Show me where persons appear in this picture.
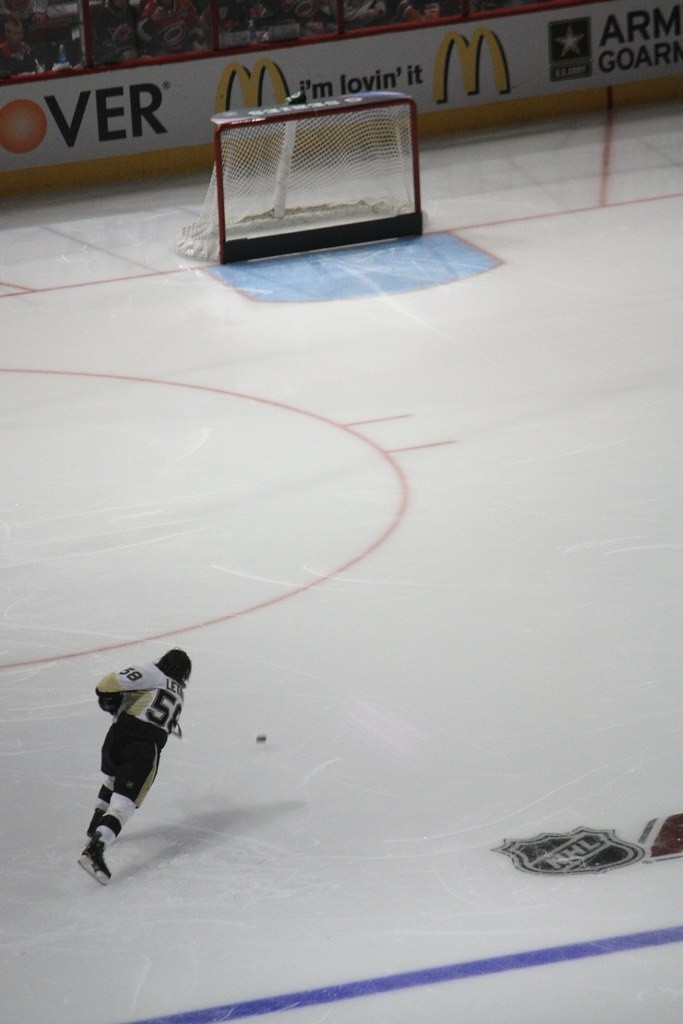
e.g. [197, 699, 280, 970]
[0, 0, 438, 76]
[78, 649, 192, 886]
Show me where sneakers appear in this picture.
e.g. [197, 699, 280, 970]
[77, 831, 112, 886]
[87, 808, 106, 838]
